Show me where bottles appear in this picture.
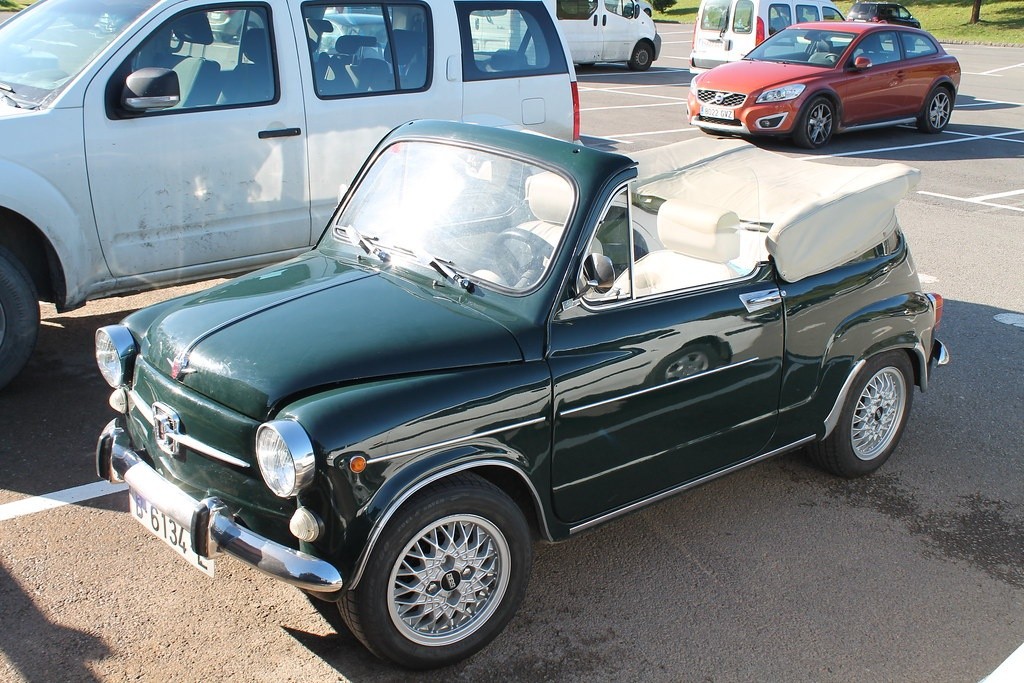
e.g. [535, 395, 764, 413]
[704, 15, 709, 27]
[738, 19, 742, 26]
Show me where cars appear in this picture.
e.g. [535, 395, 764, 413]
[307, 5, 392, 62]
[686, 21, 962, 150]
[205, 8, 263, 44]
[94, 119, 949, 671]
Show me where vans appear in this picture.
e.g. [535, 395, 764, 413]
[690, 0, 854, 74]
[469, 0, 662, 72]
[0, 1, 579, 398]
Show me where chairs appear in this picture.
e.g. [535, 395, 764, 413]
[154, 13, 222, 108]
[807, 40, 837, 65]
[606, 195, 742, 299]
[223, 26, 277, 103]
[854, 33, 884, 65]
[500, 172, 603, 281]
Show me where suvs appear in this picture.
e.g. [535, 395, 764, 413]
[846, 1, 922, 29]
